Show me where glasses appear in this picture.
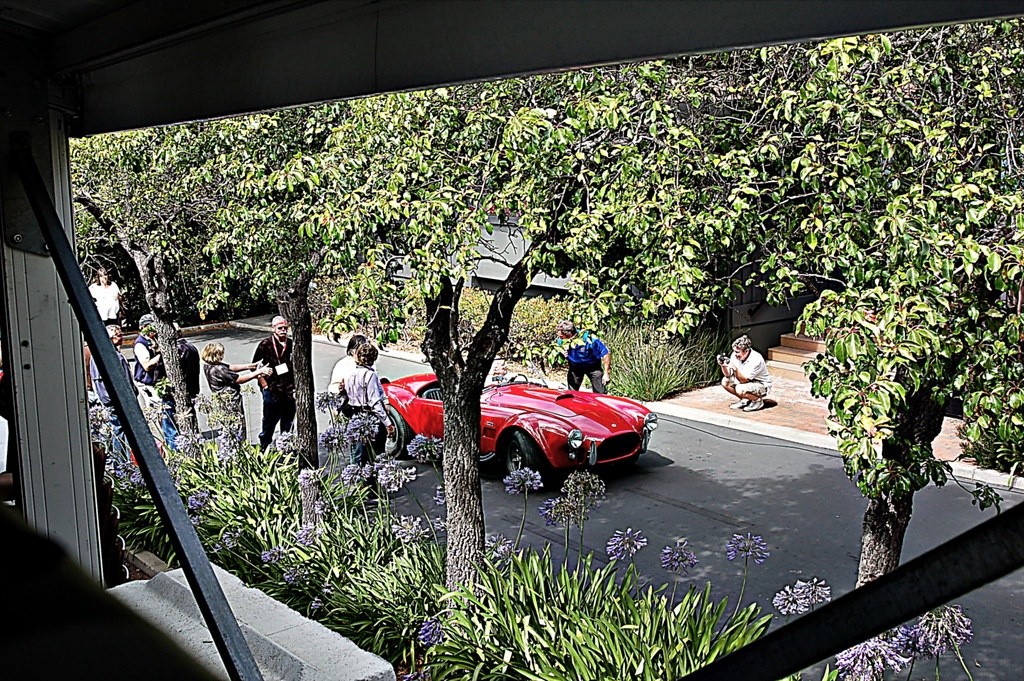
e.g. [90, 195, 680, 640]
[277, 326, 288, 329]
[556, 333, 564, 338]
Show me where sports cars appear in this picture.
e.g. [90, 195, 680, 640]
[379, 371, 658, 490]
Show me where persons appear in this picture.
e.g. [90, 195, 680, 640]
[557, 321, 610, 395]
[327, 334, 392, 414]
[88, 325, 139, 482]
[154, 323, 200, 451]
[250, 317, 297, 451]
[717, 336, 772, 412]
[87, 267, 123, 329]
[201, 342, 273, 441]
[341, 343, 397, 505]
[133, 313, 164, 445]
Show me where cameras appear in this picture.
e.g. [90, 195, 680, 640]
[718, 353, 730, 365]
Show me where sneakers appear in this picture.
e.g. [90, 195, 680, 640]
[729, 399, 750, 409]
[743, 398, 764, 412]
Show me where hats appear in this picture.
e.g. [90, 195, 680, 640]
[106, 325, 125, 339]
[139, 314, 155, 325]
[557, 322, 576, 332]
[272, 316, 288, 328]
[733, 335, 751, 350]
